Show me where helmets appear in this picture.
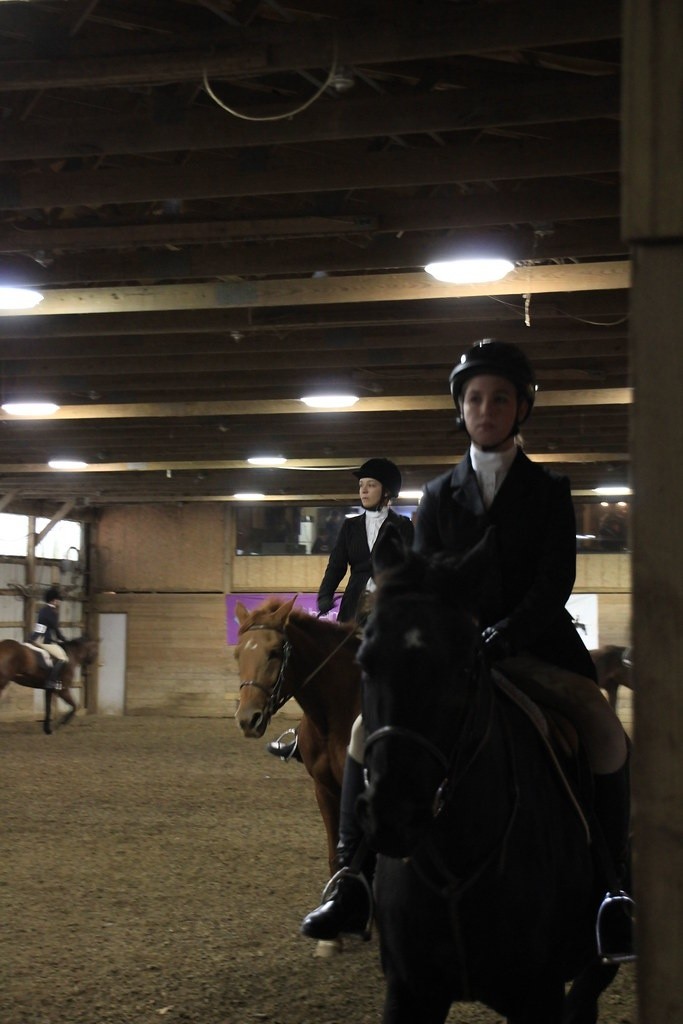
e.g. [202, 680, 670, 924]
[450, 339, 537, 421]
[46, 588, 62, 602]
[353, 458, 401, 497]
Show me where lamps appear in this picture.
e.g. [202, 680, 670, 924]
[1, 399, 61, 416]
[422, 257, 518, 285]
[0, 286, 44, 310]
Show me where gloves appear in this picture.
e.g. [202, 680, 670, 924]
[481, 626, 512, 658]
[319, 595, 333, 614]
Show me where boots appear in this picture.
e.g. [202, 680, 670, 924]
[590, 749, 637, 950]
[299, 747, 377, 940]
[268, 738, 303, 764]
[47, 659, 67, 690]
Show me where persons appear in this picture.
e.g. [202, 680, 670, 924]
[32, 587, 74, 691]
[269, 460, 415, 765]
[302, 336, 637, 959]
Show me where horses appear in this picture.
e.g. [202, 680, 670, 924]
[355, 519, 634, 1024]
[0, 635, 102, 735]
[233, 593, 366, 958]
